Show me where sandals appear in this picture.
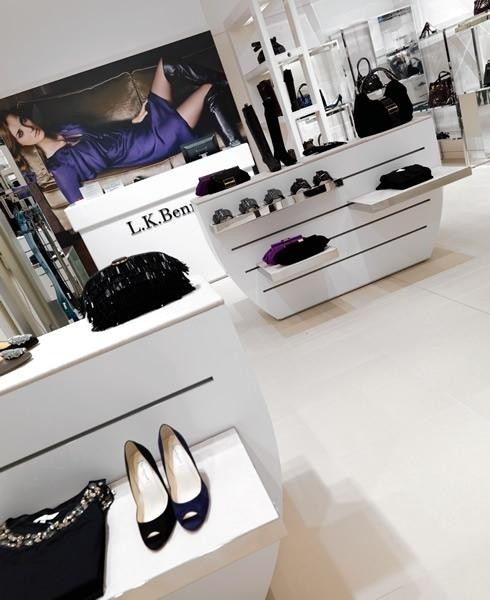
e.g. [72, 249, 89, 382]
[123, 439, 175, 550]
[157, 424, 208, 531]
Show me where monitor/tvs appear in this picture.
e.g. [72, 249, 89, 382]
[179, 132, 221, 163]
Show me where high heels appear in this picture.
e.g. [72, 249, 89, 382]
[419, 22, 437, 39]
[325, 94, 343, 111]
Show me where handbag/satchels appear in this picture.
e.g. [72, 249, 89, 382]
[80, 252, 196, 332]
[376, 164, 433, 190]
[474, 0, 489, 15]
[429, 77, 459, 108]
[263, 235, 330, 266]
[296, 89, 327, 118]
[354, 79, 413, 138]
[195, 166, 250, 197]
[258, 41, 285, 64]
[0, 479, 105, 598]
[484, 63, 490, 86]
[303, 142, 344, 156]
[287, 139, 315, 162]
[408, 60, 423, 77]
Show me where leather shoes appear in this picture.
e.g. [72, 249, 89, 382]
[0, 333, 39, 377]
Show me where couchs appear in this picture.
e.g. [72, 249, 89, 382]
[16, 47, 247, 235]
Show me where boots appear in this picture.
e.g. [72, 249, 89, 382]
[241, 105, 297, 172]
[203, 84, 244, 147]
[161, 52, 231, 97]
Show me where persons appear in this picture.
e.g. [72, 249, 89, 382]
[1, 53, 240, 206]
